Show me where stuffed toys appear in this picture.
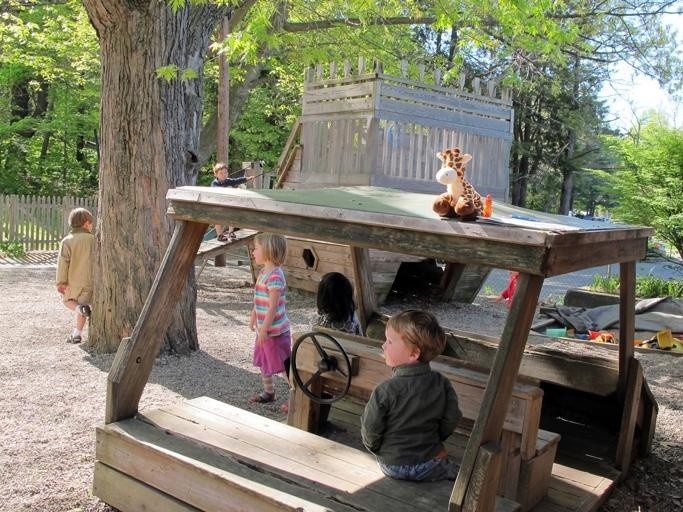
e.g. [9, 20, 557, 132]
[435, 147, 493, 218]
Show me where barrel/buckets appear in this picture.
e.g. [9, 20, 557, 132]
[545, 325, 568, 338]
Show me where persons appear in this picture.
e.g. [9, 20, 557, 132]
[360, 310, 463, 483]
[250, 232, 294, 412]
[210, 162, 254, 240]
[56, 207, 95, 343]
[283, 272, 364, 393]
[495, 267, 520, 311]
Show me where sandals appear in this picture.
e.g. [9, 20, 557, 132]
[229, 232, 236, 239]
[67, 334, 82, 344]
[252, 389, 276, 403]
[217, 234, 228, 241]
[79, 304, 90, 317]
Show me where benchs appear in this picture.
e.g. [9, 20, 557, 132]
[95, 394, 523, 511]
[286, 324, 560, 511]
